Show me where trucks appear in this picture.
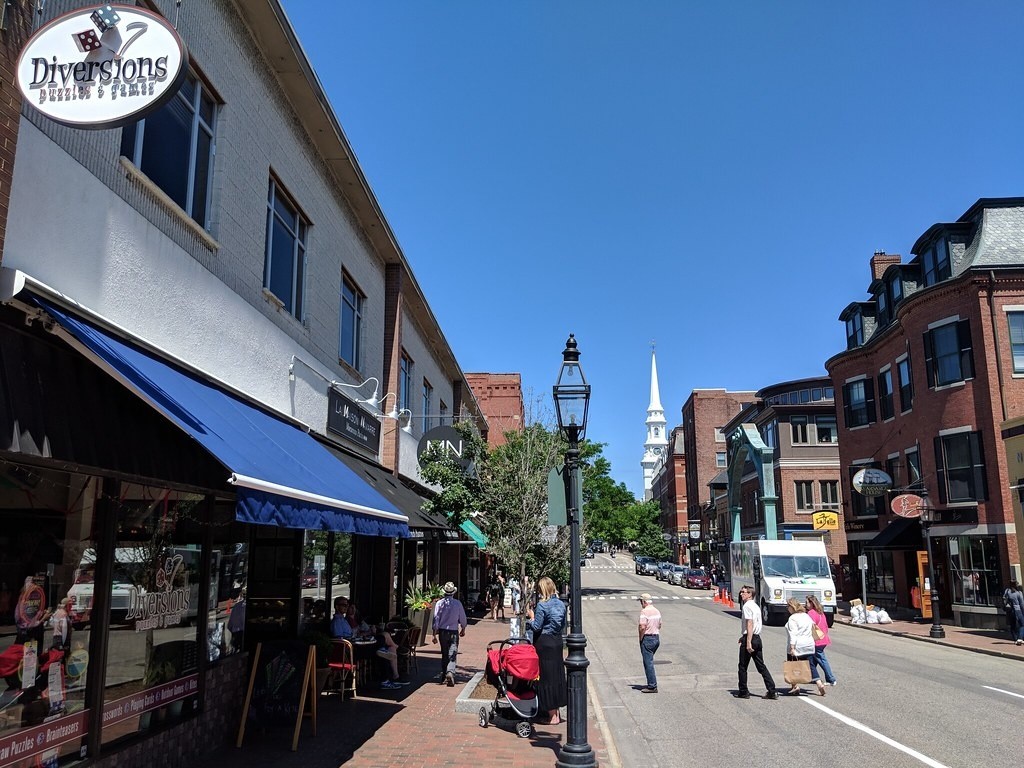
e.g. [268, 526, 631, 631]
[730, 539, 837, 628]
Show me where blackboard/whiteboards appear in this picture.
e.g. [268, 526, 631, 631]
[242, 642, 317, 717]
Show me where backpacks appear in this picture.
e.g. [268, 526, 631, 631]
[1003, 588, 1013, 612]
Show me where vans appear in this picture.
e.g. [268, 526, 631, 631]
[65, 546, 245, 632]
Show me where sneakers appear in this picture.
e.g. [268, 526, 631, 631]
[396, 647, 410, 655]
[392, 677, 410, 685]
[376, 648, 393, 660]
[381, 680, 402, 689]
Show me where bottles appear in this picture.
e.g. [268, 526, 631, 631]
[371, 625, 377, 634]
[351, 630, 355, 639]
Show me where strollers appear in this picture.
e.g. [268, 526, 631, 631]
[479, 637, 541, 738]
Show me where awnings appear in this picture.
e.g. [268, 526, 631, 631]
[317, 440, 460, 540]
[31, 293, 411, 539]
[446, 511, 490, 548]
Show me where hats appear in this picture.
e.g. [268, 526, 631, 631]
[637, 593, 652, 601]
[60, 597, 71, 608]
[442, 582, 457, 594]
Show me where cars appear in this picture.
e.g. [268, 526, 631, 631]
[681, 569, 712, 590]
[580, 538, 604, 567]
[667, 564, 689, 585]
[633, 552, 677, 581]
[302, 561, 345, 588]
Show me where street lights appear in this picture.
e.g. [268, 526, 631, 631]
[551, 332, 592, 768]
[917, 484, 945, 638]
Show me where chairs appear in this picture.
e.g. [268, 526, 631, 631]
[324, 637, 356, 702]
[385, 620, 421, 674]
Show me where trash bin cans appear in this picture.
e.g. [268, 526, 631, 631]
[718, 582, 730, 598]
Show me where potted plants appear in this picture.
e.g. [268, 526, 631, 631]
[402, 577, 432, 646]
[422, 582, 446, 634]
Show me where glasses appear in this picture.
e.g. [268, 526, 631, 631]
[337, 604, 349, 607]
[739, 590, 748, 593]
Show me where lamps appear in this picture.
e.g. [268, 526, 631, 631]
[373, 408, 413, 436]
[330, 376, 379, 408]
[355, 392, 398, 420]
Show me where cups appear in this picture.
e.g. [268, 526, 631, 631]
[379, 623, 385, 632]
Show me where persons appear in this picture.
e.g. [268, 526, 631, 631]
[509, 574, 521, 615]
[432, 582, 467, 687]
[732, 586, 779, 700]
[345, 600, 410, 685]
[228, 586, 247, 652]
[232, 576, 243, 602]
[805, 595, 836, 686]
[331, 596, 402, 688]
[784, 597, 826, 696]
[711, 564, 718, 584]
[1002, 578, 1024, 646]
[525, 577, 568, 725]
[700, 564, 704, 570]
[636, 593, 662, 693]
[53, 598, 74, 654]
[15, 575, 52, 656]
[301, 597, 325, 623]
[486, 571, 505, 620]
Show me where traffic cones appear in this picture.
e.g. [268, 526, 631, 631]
[714, 588, 734, 608]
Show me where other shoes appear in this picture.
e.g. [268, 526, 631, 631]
[540, 718, 560, 724]
[446, 672, 454, 687]
[641, 688, 658, 693]
[1015, 639, 1024, 645]
[824, 681, 836, 686]
[762, 692, 779, 699]
[734, 690, 750, 698]
[788, 687, 800, 695]
[819, 684, 825, 696]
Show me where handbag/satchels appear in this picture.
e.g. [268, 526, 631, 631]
[784, 652, 812, 684]
[811, 623, 825, 640]
